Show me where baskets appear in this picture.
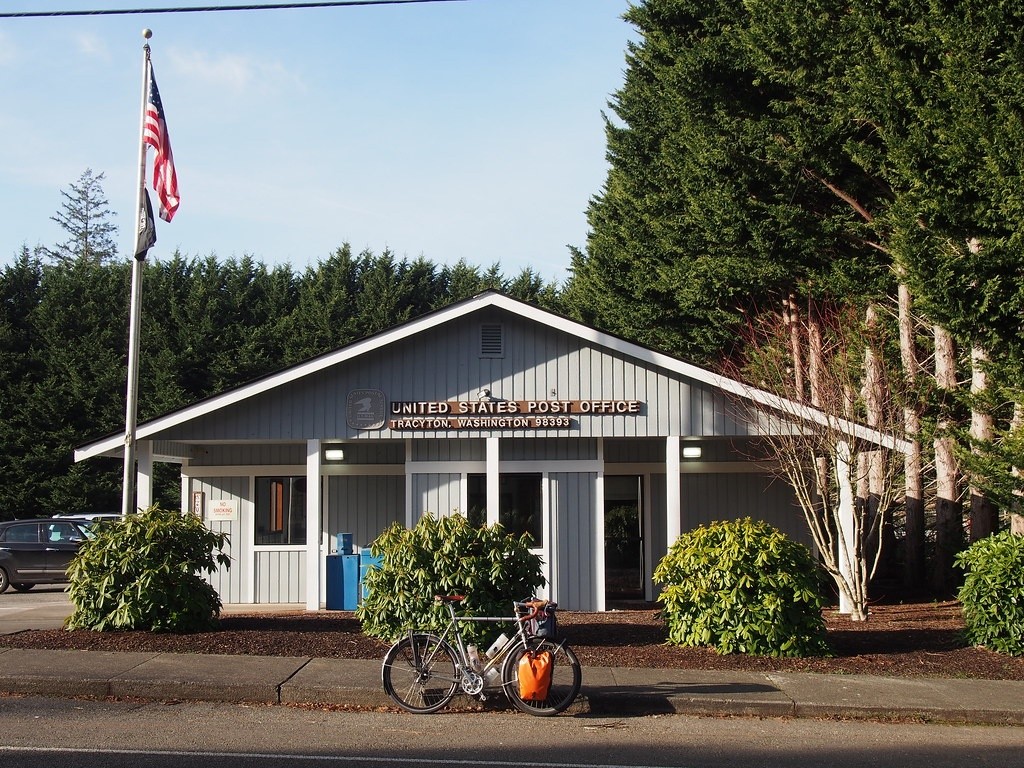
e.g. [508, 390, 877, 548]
[530, 602, 557, 638]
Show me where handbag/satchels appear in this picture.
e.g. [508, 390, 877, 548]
[517, 649, 551, 700]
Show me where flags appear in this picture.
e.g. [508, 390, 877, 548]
[131, 43, 180, 265]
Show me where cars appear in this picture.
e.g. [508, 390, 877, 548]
[0, 518, 101, 594]
[47, 511, 123, 544]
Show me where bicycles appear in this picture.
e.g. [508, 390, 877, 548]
[380, 594, 584, 718]
